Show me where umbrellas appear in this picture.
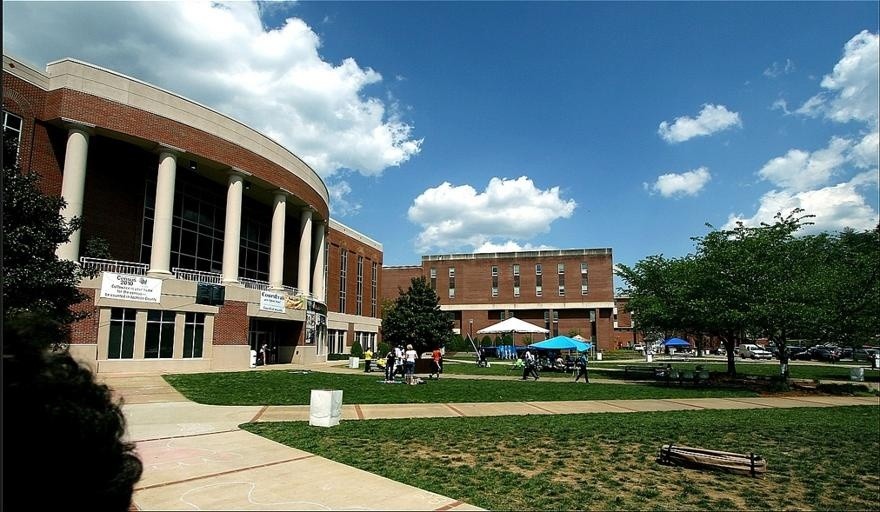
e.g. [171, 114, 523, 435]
[476, 316, 551, 347]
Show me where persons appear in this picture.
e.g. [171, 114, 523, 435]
[666, 363, 674, 377]
[393, 341, 406, 378]
[364, 346, 374, 372]
[400, 343, 419, 386]
[520, 348, 539, 380]
[495, 344, 518, 360]
[384, 348, 396, 382]
[574, 350, 590, 384]
[259, 343, 268, 366]
[429, 347, 442, 380]
[532, 353, 581, 374]
[656, 363, 667, 377]
[0, 339, 144, 512]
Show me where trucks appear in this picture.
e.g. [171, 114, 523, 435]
[738, 343, 773, 360]
